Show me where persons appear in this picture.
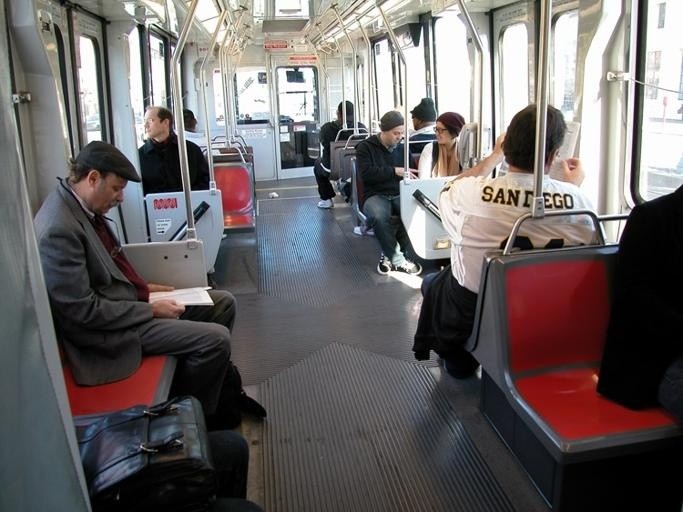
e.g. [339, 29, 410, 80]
[416, 111, 463, 180]
[355, 108, 424, 277]
[351, 97, 438, 237]
[595, 183, 682, 427]
[313, 99, 368, 210]
[33, 140, 266, 430]
[419, 101, 606, 379]
[203, 430, 264, 512]
[183, 108, 221, 155]
[138, 105, 211, 194]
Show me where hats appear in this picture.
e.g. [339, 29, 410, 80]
[77, 140, 142, 183]
[436, 112, 466, 136]
[410, 98, 437, 123]
[381, 111, 405, 132]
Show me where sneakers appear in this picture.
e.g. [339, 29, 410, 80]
[352, 226, 374, 236]
[393, 249, 423, 275]
[317, 199, 335, 209]
[377, 251, 392, 275]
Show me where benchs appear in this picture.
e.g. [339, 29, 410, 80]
[141, 135, 255, 234]
[319, 129, 472, 235]
[61, 355, 178, 426]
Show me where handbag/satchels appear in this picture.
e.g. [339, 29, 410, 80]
[209, 362, 246, 431]
[73, 395, 216, 508]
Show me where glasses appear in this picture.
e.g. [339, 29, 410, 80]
[433, 127, 448, 135]
[100, 215, 122, 260]
